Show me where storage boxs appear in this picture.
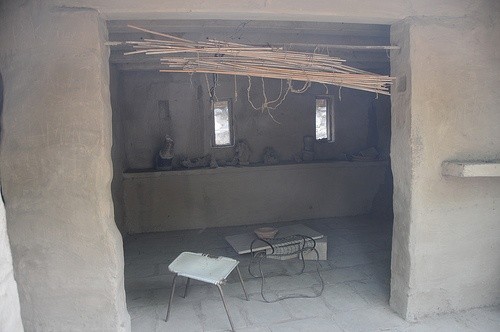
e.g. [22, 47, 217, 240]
[265, 243, 300, 261]
[298, 234, 328, 261]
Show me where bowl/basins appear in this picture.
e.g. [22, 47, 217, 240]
[256, 227, 277, 239]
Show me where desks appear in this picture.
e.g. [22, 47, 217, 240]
[248, 234, 325, 302]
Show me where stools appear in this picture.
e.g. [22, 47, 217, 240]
[163, 250, 248, 332]
